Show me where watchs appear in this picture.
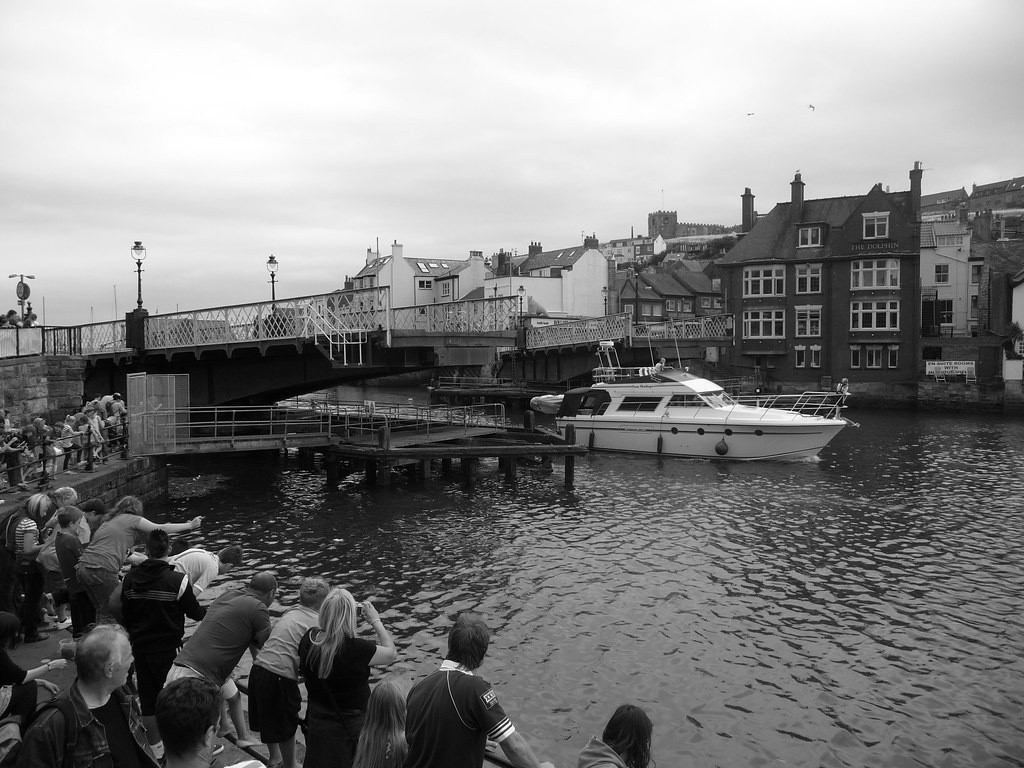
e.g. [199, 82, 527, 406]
[47, 662, 51, 671]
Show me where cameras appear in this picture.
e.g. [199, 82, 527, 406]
[356, 607, 364, 615]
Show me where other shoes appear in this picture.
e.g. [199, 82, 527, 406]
[104, 461, 108, 465]
[93, 453, 100, 459]
[213, 743, 224, 756]
[216, 726, 236, 739]
[39, 633, 50, 640]
[56, 619, 72, 631]
[43, 614, 57, 623]
[236, 739, 263, 748]
[64, 470, 71, 475]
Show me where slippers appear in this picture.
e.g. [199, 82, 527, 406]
[267, 756, 284, 768]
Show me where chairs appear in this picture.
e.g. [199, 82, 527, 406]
[934, 367, 947, 383]
[639, 367, 654, 377]
[965, 367, 977, 384]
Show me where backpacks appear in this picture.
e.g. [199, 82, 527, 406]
[105, 401, 120, 418]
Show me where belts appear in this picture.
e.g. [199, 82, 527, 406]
[173, 662, 191, 669]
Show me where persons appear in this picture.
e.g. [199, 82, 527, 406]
[654, 357, 666, 372]
[836, 377, 849, 407]
[247, 576, 330, 768]
[0, 485, 107, 644]
[399, 616, 555, 768]
[298, 587, 398, 768]
[120, 529, 208, 768]
[0, 309, 41, 328]
[155, 676, 224, 768]
[13, 623, 161, 768]
[0, 392, 163, 505]
[74, 496, 202, 623]
[577, 702, 654, 768]
[0, 609, 68, 735]
[350, 676, 407, 768]
[162, 572, 278, 748]
[107, 538, 243, 641]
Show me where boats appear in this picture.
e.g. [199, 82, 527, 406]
[528, 339, 852, 465]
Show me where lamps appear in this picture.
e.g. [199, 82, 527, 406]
[754, 388, 761, 395]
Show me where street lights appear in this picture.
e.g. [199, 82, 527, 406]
[266, 254, 278, 311]
[132, 241, 147, 311]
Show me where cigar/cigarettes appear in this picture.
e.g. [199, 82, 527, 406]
[200, 517, 204, 520]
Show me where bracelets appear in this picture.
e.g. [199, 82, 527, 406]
[369, 618, 381, 626]
